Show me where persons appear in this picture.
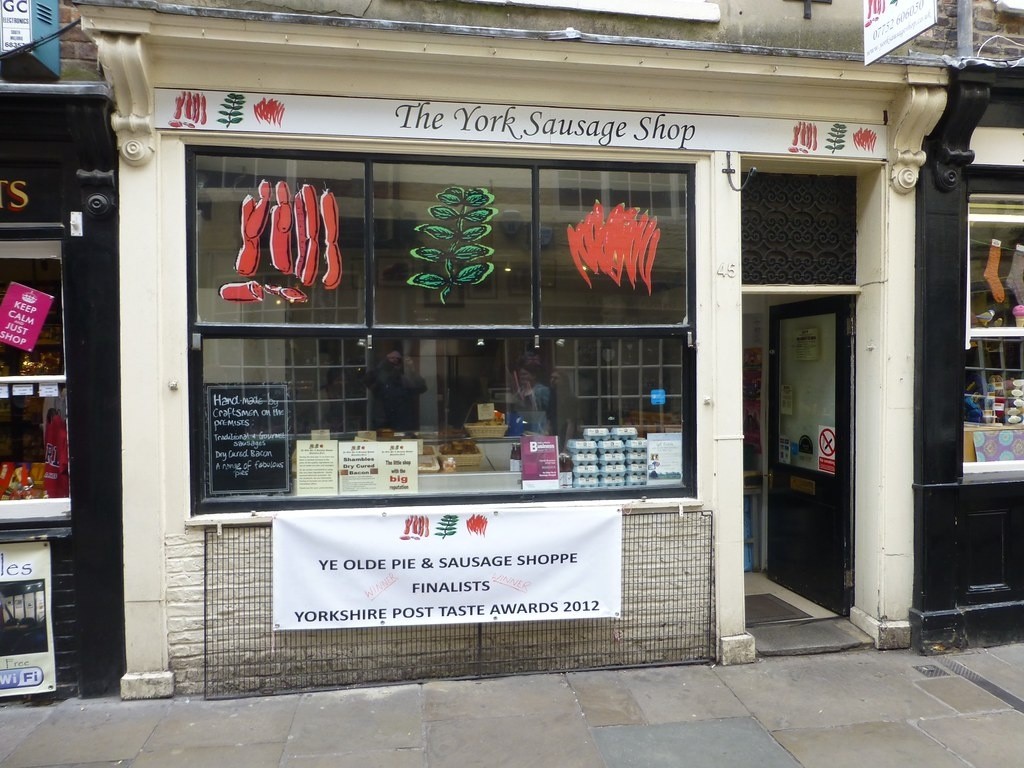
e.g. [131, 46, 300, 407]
[361, 350, 428, 439]
[510, 352, 584, 473]
